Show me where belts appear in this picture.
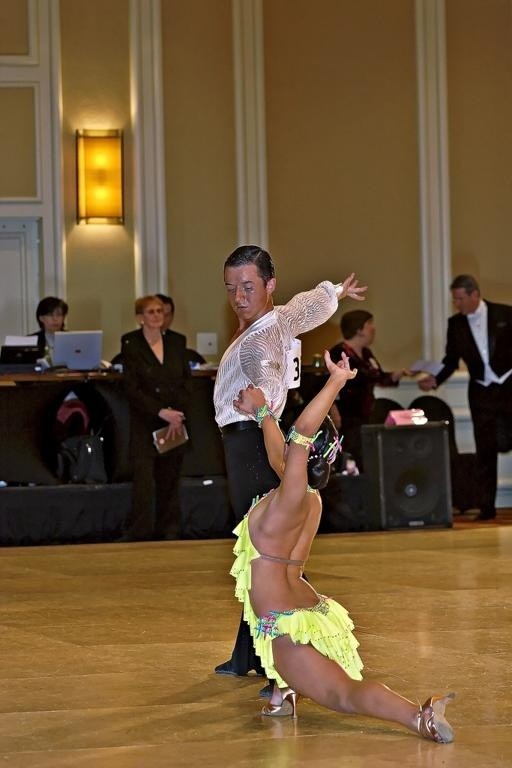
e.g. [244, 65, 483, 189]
[221, 422, 258, 437]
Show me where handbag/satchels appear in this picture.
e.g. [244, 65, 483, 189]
[50, 427, 108, 483]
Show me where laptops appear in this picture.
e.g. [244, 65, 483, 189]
[52, 330, 103, 372]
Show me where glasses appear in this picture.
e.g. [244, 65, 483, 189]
[143, 309, 163, 313]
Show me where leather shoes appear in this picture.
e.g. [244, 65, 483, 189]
[260, 684, 274, 697]
[215, 659, 263, 677]
[474, 507, 497, 521]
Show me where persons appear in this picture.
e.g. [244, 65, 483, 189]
[229, 345, 458, 743]
[23, 295, 114, 371]
[107, 293, 206, 372]
[212, 244, 369, 697]
[123, 293, 194, 541]
[323, 304, 420, 447]
[418, 267, 512, 523]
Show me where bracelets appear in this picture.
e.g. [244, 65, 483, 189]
[247, 405, 280, 421]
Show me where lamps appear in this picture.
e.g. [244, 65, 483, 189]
[73, 128, 124, 224]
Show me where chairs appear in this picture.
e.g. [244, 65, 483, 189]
[372, 395, 478, 518]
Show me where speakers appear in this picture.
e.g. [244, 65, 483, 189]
[179, 478, 234, 538]
[358, 421, 456, 530]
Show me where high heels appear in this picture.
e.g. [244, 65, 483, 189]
[258, 686, 299, 718]
[417, 693, 455, 744]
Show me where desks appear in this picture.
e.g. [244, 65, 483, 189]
[2, 364, 330, 486]
